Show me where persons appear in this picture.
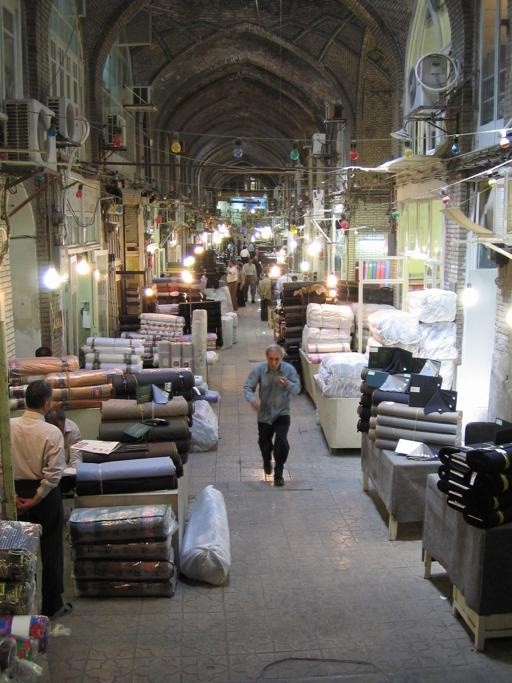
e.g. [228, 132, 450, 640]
[32, 344, 53, 357]
[44, 403, 84, 467]
[243, 341, 302, 487]
[214, 234, 318, 321]
[8, 377, 76, 621]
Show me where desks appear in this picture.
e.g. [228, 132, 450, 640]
[359, 432, 512, 652]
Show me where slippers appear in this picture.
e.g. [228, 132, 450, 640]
[48, 603, 73, 620]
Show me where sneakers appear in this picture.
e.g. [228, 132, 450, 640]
[263, 460, 272, 474]
[274, 478, 284, 487]
